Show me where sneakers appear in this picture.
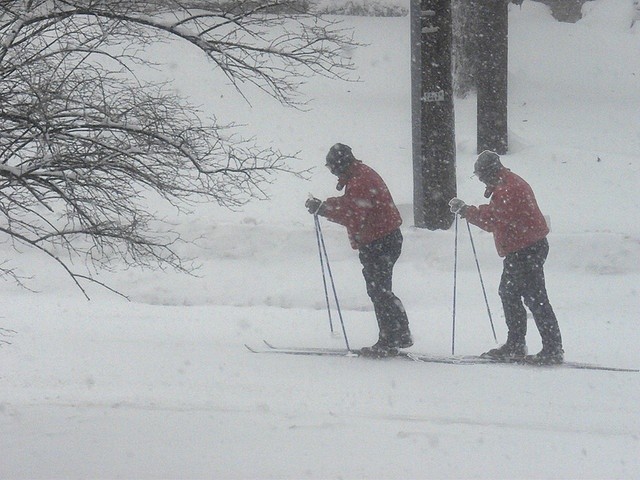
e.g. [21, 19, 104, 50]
[361, 332, 398, 358]
[379, 329, 413, 347]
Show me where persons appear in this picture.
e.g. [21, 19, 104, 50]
[305, 143, 413, 355]
[448, 150, 564, 365]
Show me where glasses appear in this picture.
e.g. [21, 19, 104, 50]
[326, 143, 354, 178]
[325, 163, 334, 174]
[473, 170, 484, 177]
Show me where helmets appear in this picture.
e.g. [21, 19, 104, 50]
[474, 150, 502, 186]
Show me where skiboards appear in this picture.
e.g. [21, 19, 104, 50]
[244, 340, 416, 360]
[419, 356, 635, 374]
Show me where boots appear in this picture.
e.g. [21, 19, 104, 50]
[487, 331, 525, 357]
[525, 340, 564, 365]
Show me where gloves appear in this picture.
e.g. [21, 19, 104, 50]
[305, 198, 326, 216]
[448, 197, 468, 215]
